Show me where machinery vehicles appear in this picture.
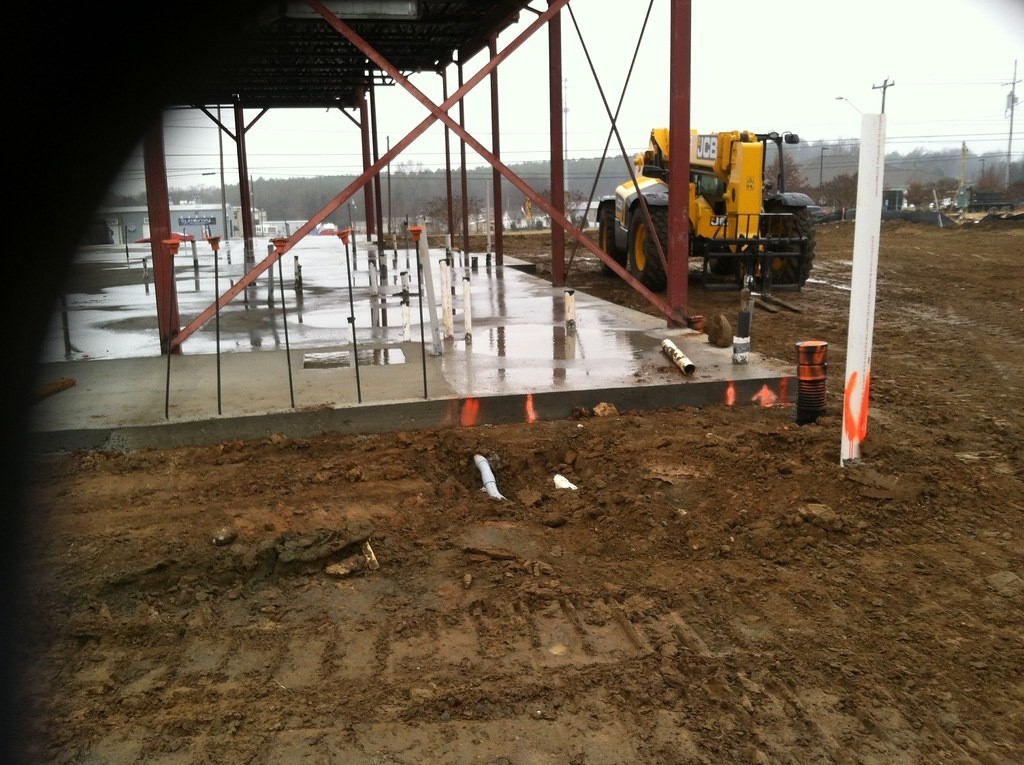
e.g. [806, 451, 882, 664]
[596, 128, 816, 293]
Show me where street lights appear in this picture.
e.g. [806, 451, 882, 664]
[835, 96, 863, 114]
[820, 148, 828, 185]
[978, 159, 985, 178]
